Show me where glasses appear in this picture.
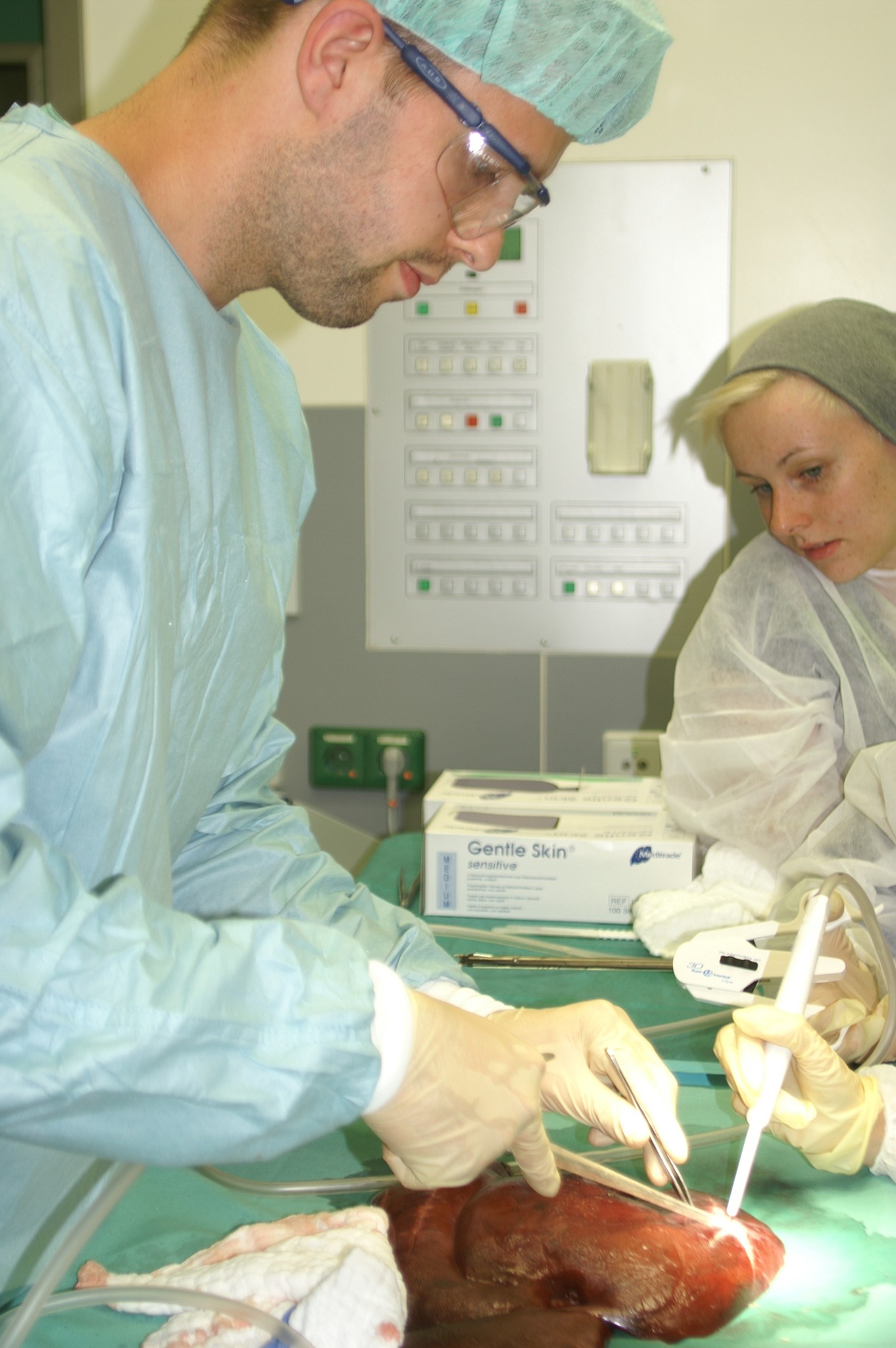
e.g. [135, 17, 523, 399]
[281, 0, 551, 240]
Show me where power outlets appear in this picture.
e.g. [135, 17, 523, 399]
[308, 726, 363, 790]
[602, 730, 664, 777]
[362, 728, 425, 793]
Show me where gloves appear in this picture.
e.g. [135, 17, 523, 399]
[806, 889, 896, 1065]
[362, 988, 561, 1198]
[486, 999, 688, 1188]
[712, 1006, 884, 1175]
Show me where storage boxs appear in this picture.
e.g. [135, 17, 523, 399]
[422, 803, 696, 925]
[421, 769, 666, 828]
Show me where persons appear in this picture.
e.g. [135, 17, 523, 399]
[2, 1, 693, 1202]
[660, 298, 896, 1180]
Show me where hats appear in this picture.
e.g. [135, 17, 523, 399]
[722, 299, 896, 444]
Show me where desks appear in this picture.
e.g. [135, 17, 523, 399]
[1, 832, 896, 1348]
